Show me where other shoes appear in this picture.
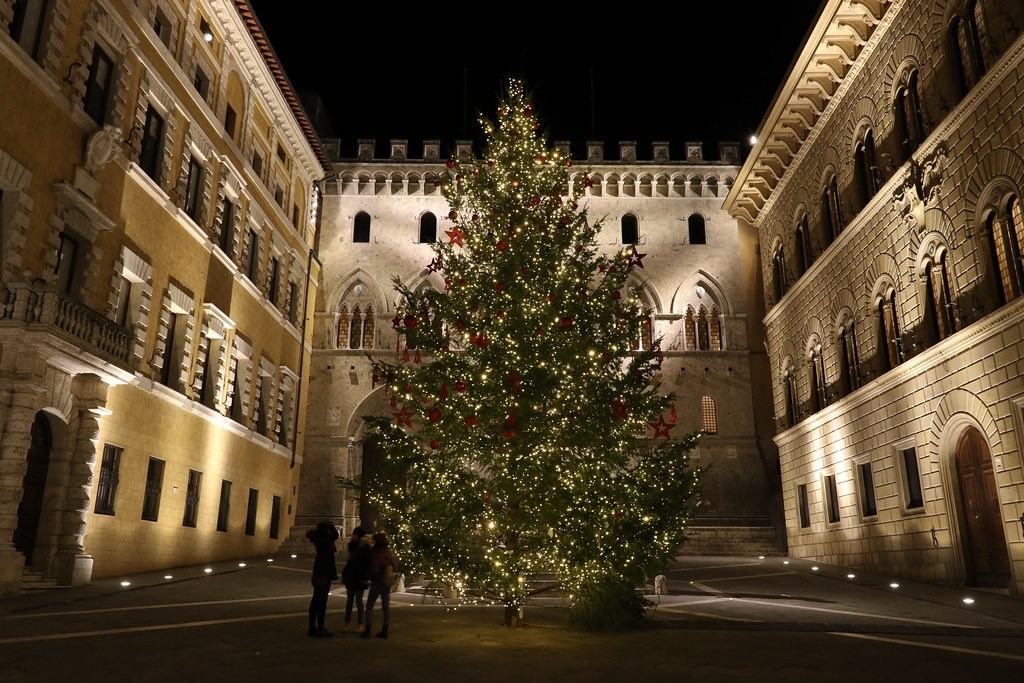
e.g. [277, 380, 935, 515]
[316, 629, 335, 636]
[355, 628, 364, 634]
[308, 629, 317, 637]
[344, 628, 349, 633]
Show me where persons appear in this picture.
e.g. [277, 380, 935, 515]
[342, 526, 371, 633]
[360, 533, 392, 639]
[305, 522, 339, 637]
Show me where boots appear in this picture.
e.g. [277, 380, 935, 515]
[375, 623, 389, 639]
[360, 624, 372, 638]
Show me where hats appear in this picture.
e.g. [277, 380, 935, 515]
[352, 526, 365, 536]
[373, 532, 393, 545]
